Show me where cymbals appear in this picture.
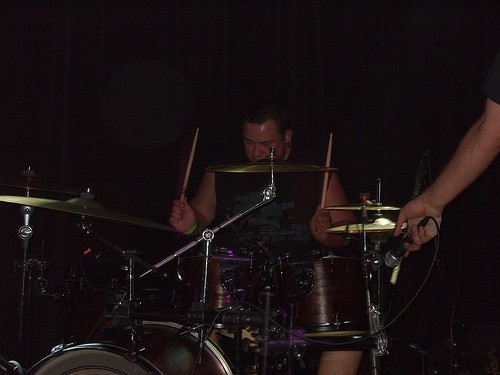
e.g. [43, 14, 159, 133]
[0, 194, 178, 234]
[324, 205, 401, 210]
[324, 218, 408, 235]
[0, 179, 63, 193]
[203, 160, 343, 174]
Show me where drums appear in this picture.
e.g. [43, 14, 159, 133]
[25, 319, 233, 375]
[175, 242, 280, 330]
[13, 258, 89, 302]
[277, 245, 375, 342]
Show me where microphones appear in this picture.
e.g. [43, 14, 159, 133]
[384, 217, 430, 267]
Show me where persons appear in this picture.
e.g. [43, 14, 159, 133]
[393, 48, 499, 258]
[169, 99, 366, 375]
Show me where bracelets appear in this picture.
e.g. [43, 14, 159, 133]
[184, 222, 202, 235]
